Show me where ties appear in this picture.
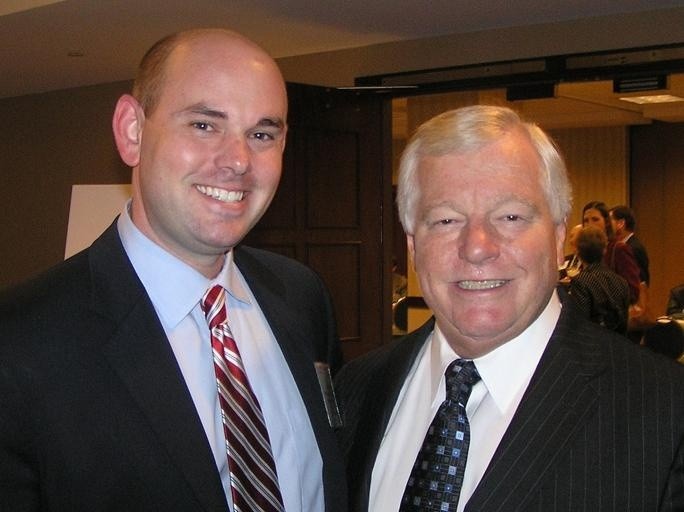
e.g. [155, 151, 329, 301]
[398, 359, 481, 511]
[200, 285, 286, 512]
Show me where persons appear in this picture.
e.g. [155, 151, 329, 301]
[0, 26, 343, 511]
[391, 256, 408, 302]
[329, 97, 683, 511]
[556, 200, 683, 365]
